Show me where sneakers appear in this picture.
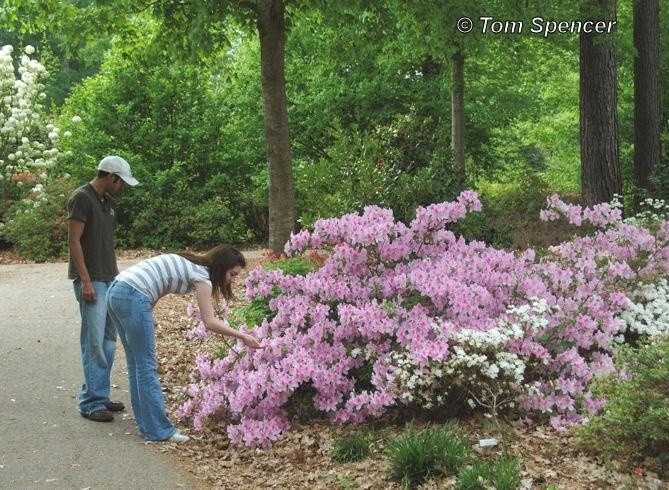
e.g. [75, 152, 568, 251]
[106, 400, 125, 412]
[79, 408, 114, 422]
[168, 428, 191, 444]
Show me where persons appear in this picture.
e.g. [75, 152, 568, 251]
[105, 244, 265, 444]
[65, 156, 140, 422]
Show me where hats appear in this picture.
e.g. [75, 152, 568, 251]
[97, 155, 140, 186]
